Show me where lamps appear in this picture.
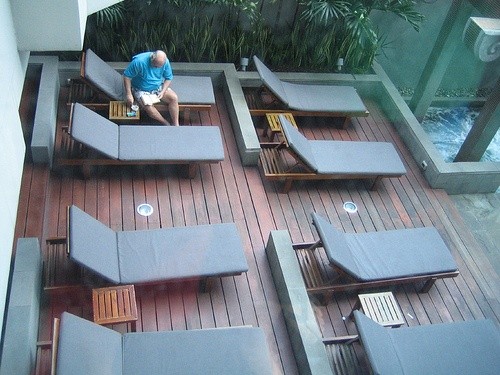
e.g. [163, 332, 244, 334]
[335, 57, 344, 71]
[239, 58, 249, 70]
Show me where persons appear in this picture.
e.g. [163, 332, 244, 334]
[123, 50, 179, 126]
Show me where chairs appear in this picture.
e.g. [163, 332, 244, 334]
[44, 205, 248, 292]
[248, 55, 369, 130]
[322, 310, 500, 375]
[259, 114, 406, 194]
[34, 311, 274, 375]
[66, 49, 216, 122]
[292, 211, 460, 305]
[57, 103, 225, 179]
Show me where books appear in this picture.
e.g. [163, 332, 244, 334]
[141, 94, 160, 105]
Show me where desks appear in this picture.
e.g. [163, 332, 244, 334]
[348, 291, 406, 328]
[92, 284, 139, 333]
[262, 111, 298, 143]
[108, 101, 141, 125]
